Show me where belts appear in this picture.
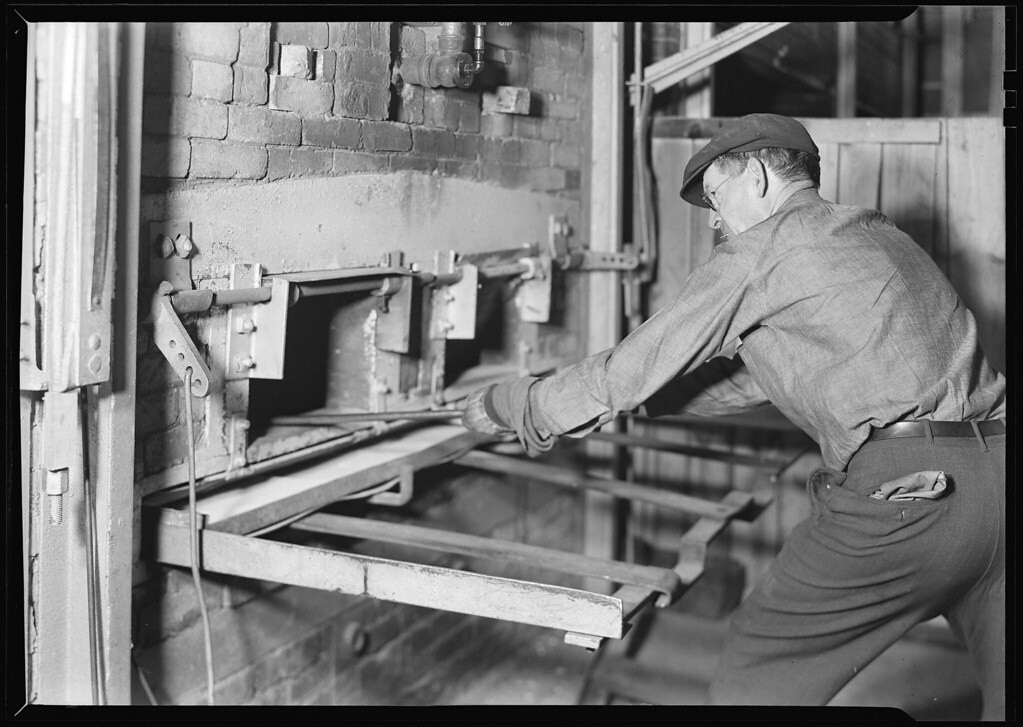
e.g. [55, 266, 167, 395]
[864, 417, 1005, 443]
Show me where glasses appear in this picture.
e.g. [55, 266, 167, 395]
[698, 155, 763, 212]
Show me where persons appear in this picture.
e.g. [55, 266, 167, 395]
[462, 112, 1006, 722]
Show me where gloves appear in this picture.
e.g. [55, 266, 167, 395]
[455, 383, 517, 437]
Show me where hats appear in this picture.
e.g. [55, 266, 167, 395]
[680, 113, 820, 209]
